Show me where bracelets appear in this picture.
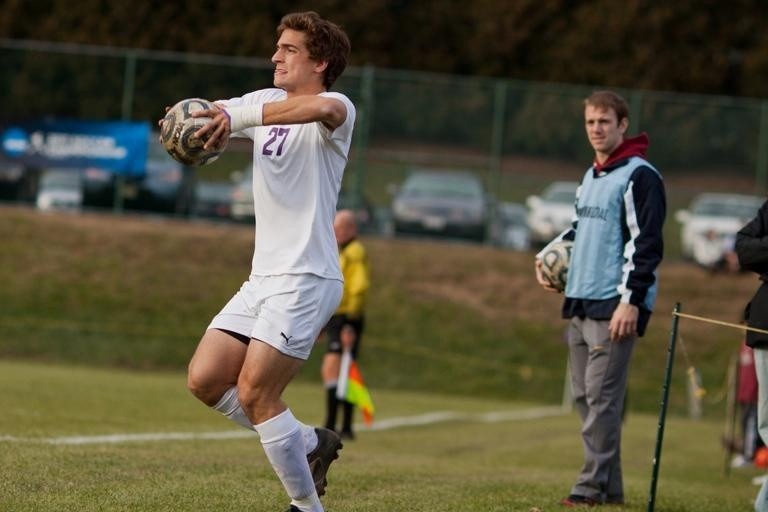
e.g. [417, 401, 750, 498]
[229, 106, 264, 134]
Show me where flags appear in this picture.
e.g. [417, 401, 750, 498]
[336, 349, 378, 429]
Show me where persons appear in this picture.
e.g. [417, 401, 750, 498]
[321, 209, 370, 441]
[729, 320, 757, 467]
[733, 199, 768, 512]
[535, 91, 667, 507]
[695, 231, 729, 270]
[157, 12, 355, 511]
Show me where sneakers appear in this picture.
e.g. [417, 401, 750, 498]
[559, 494, 603, 508]
[305, 427, 344, 500]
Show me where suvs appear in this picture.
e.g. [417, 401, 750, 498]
[385, 166, 500, 242]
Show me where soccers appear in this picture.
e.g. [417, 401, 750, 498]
[540, 241, 574, 293]
[161, 97, 223, 165]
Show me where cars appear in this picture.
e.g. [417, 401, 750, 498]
[526, 181, 581, 250]
[4, 134, 257, 227]
[674, 192, 767, 267]
[336, 190, 379, 238]
[488, 198, 532, 253]
